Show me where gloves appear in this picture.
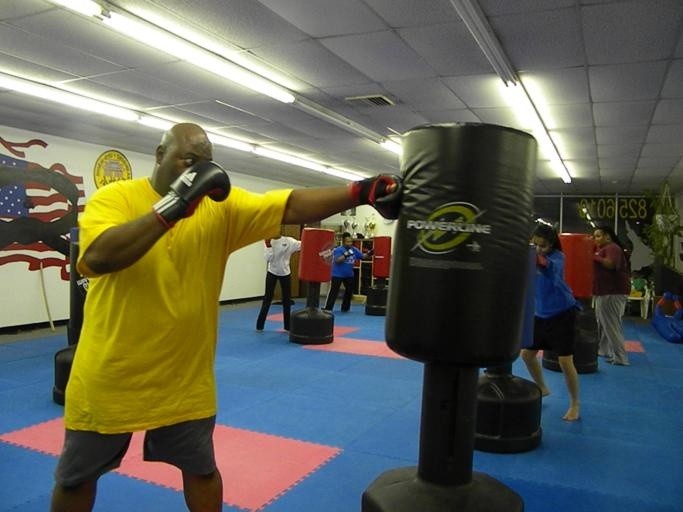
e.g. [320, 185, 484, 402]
[346, 169, 404, 220]
[368, 246, 375, 258]
[263, 237, 273, 248]
[343, 247, 354, 262]
[148, 157, 232, 233]
[536, 255, 547, 269]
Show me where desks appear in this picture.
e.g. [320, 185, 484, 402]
[592, 295, 645, 319]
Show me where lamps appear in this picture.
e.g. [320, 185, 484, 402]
[51, 0, 104, 19]
[450, 0, 518, 87]
[252, 146, 326, 173]
[136, 113, 255, 153]
[504, 82, 571, 184]
[382, 140, 403, 156]
[100, 8, 295, 104]
[322, 167, 365, 182]
[289, 96, 387, 144]
[1, 73, 140, 123]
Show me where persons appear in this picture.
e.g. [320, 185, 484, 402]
[591, 226, 630, 366]
[49, 122, 402, 511]
[256, 235, 300, 334]
[518, 225, 580, 423]
[325, 231, 375, 311]
